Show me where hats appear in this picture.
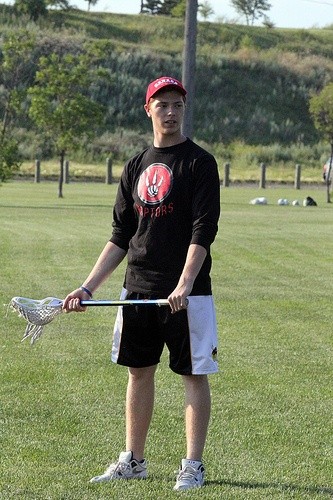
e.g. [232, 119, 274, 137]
[146, 76, 187, 105]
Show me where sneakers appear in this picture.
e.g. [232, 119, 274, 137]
[172, 458, 205, 492]
[89, 450, 147, 484]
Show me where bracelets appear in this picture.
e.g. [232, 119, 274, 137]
[81, 286, 92, 299]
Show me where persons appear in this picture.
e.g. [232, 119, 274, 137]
[61, 77, 221, 491]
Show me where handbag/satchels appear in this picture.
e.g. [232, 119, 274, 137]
[303, 196, 317, 207]
